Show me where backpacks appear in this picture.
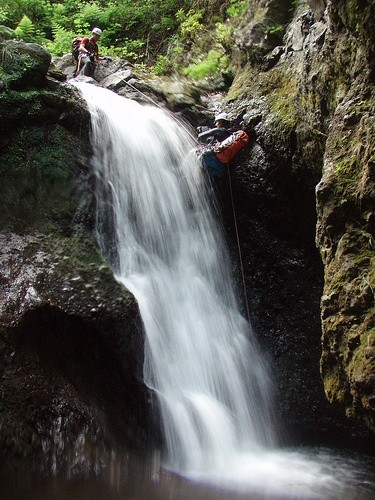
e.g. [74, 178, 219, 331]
[71, 35, 92, 64]
[212, 128, 250, 164]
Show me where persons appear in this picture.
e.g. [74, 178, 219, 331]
[76, 28, 101, 76]
[196, 119, 231, 173]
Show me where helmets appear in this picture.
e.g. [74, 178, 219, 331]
[92, 27, 103, 36]
[213, 112, 231, 124]
